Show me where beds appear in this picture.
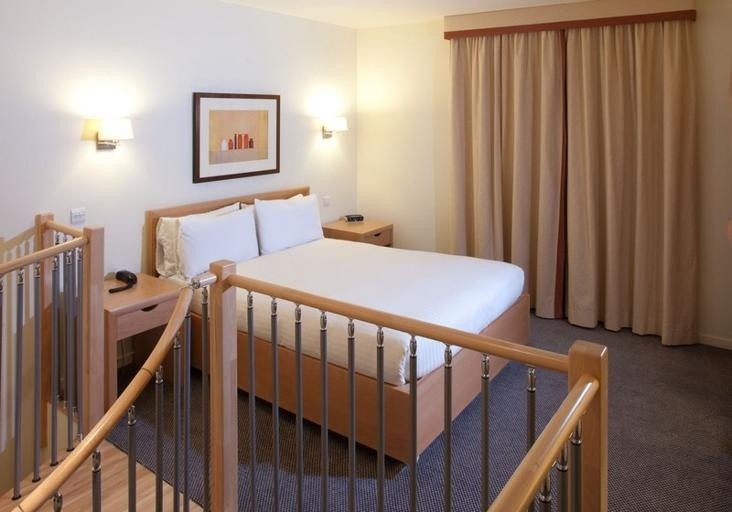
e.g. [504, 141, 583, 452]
[144, 186, 531, 466]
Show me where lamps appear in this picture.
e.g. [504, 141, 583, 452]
[82, 115, 135, 152]
[322, 117, 348, 139]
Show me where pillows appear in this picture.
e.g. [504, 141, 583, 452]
[152, 201, 241, 278]
[177, 206, 261, 279]
[253, 193, 326, 257]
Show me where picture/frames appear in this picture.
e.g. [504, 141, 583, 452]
[191, 90, 282, 183]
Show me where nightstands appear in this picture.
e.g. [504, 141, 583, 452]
[55, 272, 193, 417]
[322, 217, 395, 249]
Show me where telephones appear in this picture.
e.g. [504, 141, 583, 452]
[117, 271, 138, 285]
